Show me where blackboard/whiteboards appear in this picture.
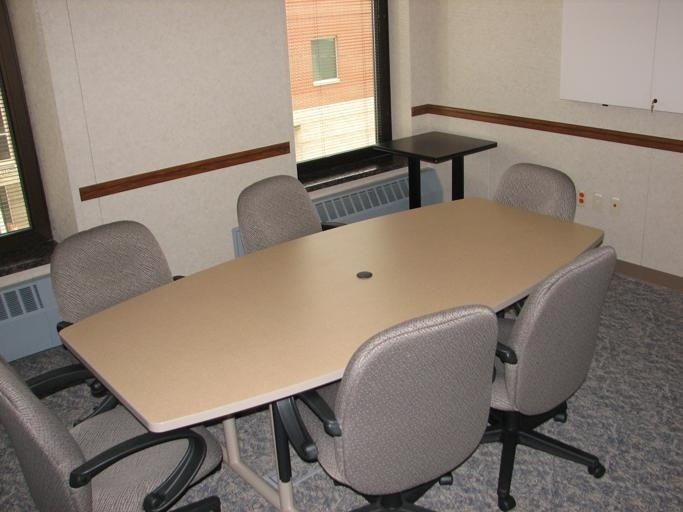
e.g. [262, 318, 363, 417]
[559, 1, 683, 113]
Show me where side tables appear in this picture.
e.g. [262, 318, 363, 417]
[374, 132, 498, 209]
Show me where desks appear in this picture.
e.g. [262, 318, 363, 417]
[55, 197, 609, 512]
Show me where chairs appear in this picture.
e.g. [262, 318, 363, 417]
[1, 356, 227, 512]
[274, 303, 505, 511]
[48, 218, 190, 428]
[433, 244, 613, 511]
[492, 164, 581, 224]
[230, 174, 347, 260]
